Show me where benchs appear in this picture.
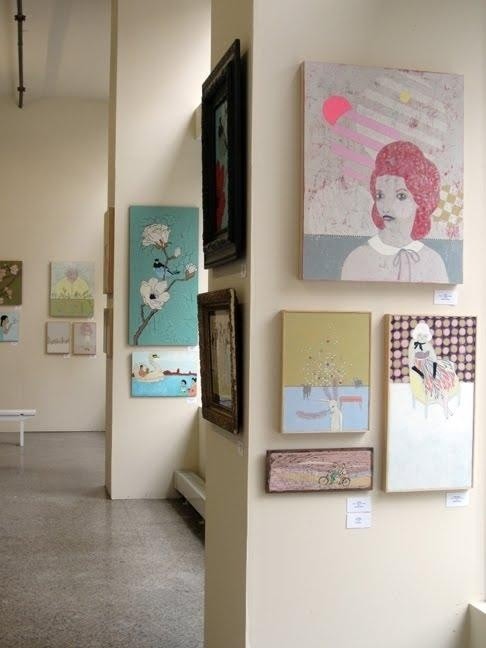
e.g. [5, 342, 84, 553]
[0, 408, 36, 446]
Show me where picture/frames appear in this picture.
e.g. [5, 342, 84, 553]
[195, 286, 243, 435]
[200, 37, 247, 270]
[264, 448, 374, 494]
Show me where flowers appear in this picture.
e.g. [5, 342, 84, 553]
[135, 224, 195, 344]
[0, 260, 20, 304]
